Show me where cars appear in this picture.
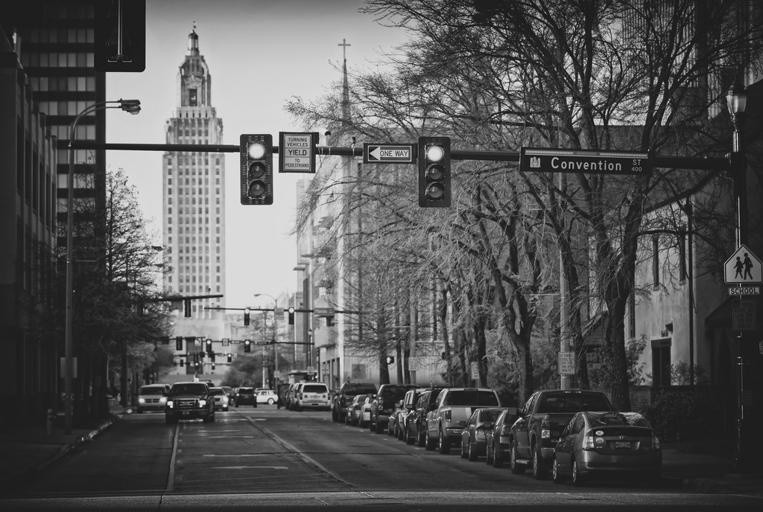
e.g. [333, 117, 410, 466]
[131, 369, 332, 422]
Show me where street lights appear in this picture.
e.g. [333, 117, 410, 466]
[55, 94, 141, 434]
[118, 237, 165, 406]
[719, 76, 760, 488]
[255, 290, 280, 388]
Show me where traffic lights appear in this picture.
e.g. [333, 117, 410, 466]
[247, 143, 266, 199]
[227, 353, 230, 362]
[211, 363, 214, 370]
[288, 307, 294, 324]
[244, 340, 250, 353]
[194, 362, 198, 371]
[423, 144, 445, 202]
[244, 308, 248, 324]
[206, 339, 211, 352]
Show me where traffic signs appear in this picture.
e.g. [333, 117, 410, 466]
[230, 340, 240, 343]
[277, 130, 319, 174]
[313, 307, 334, 315]
[516, 146, 651, 175]
[366, 144, 409, 162]
